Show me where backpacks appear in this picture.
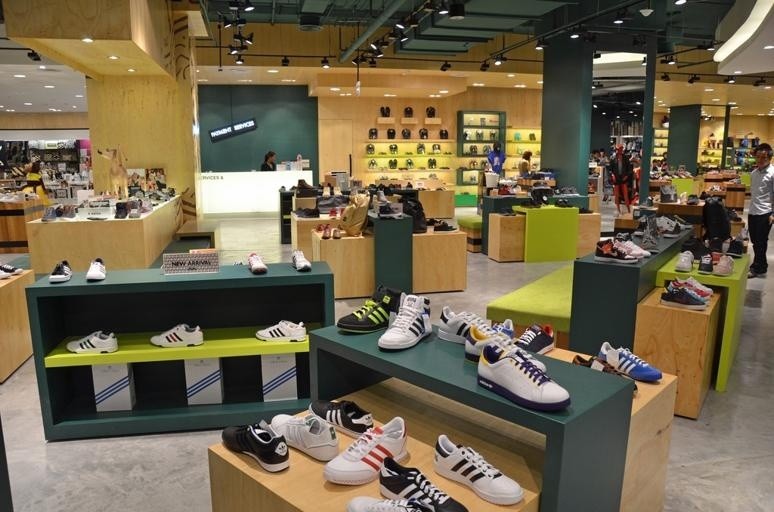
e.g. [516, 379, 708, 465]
[700, 197, 733, 242]
[398, 198, 427, 234]
[340, 190, 371, 237]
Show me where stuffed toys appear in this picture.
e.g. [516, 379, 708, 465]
[21, 162, 55, 208]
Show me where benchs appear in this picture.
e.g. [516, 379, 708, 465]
[150, 217, 220, 269]
[456, 214, 482, 253]
[486, 260, 573, 348]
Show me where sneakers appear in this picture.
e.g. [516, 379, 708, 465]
[673, 280, 710, 299]
[646, 196, 654, 209]
[393, 144, 396, 154]
[469, 161, 473, 169]
[614, 233, 632, 243]
[270, 414, 339, 462]
[473, 161, 477, 169]
[417, 144, 421, 154]
[436, 144, 439, 154]
[433, 433, 523, 504]
[568, 185, 579, 196]
[541, 190, 549, 206]
[729, 211, 742, 222]
[409, 107, 412, 117]
[86, 258, 106, 281]
[0, 271, 12, 280]
[498, 185, 504, 194]
[484, 161, 488, 169]
[387, 129, 390, 139]
[465, 318, 546, 373]
[499, 208, 509, 216]
[165, 188, 175, 197]
[426, 218, 444, 227]
[532, 181, 550, 189]
[328, 209, 337, 219]
[297, 180, 312, 189]
[476, 130, 480, 140]
[553, 188, 559, 195]
[48, 260, 72, 283]
[491, 318, 514, 339]
[726, 240, 743, 258]
[578, 208, 593, 214]
[633, 220, 647, 236]
[322, 186, 330, 198]
[747, 267, 767, 278]
[662, 222, 680, 238]
[425, 107, 432, 118]
[378, 456, 469, 512]
[709, 237, 722, 255]
[371, 159, 375, 168]
[741, 228, 749, 240]
[291, 249, 311, 271]
[509, 185, 520, 193]
[300, 208, 319, 217]
[541, 168, 553, 174]
[479, 130, 484, 140]
[221, 419, 288, 472]
[428, 159, 432, 169]
[289, 186, 297, 191]
[372, 129, 376, 139]
[430, 107, 435, 118]
[520, 200, 540, 208]
[418, 129, 423, 138]
[377, 293, 432, 349]
[560, 187, 572, 196]
[483, 145, 487, 153]
[149, 323, 204, 347]
[410, 159, 413, 169]
[655, 216, 685, 230]
[404, 107, 408, 117]
[391, 129, 395, 139]
[423, 129, 427, 138]
[615, 240, 643, 260]
[53, 205, 63, 217]
[507, 208, 516, 216]
[434, 220, 453, 229]
[321, 229, 331, 238]
[433, 224, 458, 233]
[598, 342, 661, 380]
[406, 129, 410, 139]
[389, 160, 393, 168]
[433, 144, 436, 154]
[374, 188, 392, 196]
[378, 204, 401, 218]
[698, 253, 713, 274]
[401, 130, 406, 139]
[393, 159, 397, 168]
[318, 198, 340, 210]
[368, 160, 371, 169]
[660, 285, 705, 311]
[323, 416, 407, 485]
[666, 281, 710, 304]
[0, 264, 22, 275]
[470, 146, 473, 153]
[477, 342, 570, 411]
[345, 496, 434, 511]
[278, 186, 285, 192]
[588, 183, 594, 194]
[333, 187, 341, 197]
[389, 144, 393, 154]
[368, 129, 372, 139]
[308, 399, 373, 438]
[563, 199, 573, 208]
[536, 168, 546, 174]
[385, 107, 390, 116]
[380, 106, 385, 117]
[432, 159, 435, 169]
[675, 276, 713, 295]
[693, 194, 698, 205]
[675, 251, 694, 272]
[255, 320, 305, 342]
[713, 255, 734, 275]
[513, 322, 553, 354]
[572, 354, 638, 394]
[488, 145, 491, 153]
[474, 145, 477, 154]
[370, 144, 374, 154]
[594, 239, 638, 263]
[406, 160, 410, 169]
[421, 144, 424, 154]
[531, 191, 542, 205]
[555, 199, 566, 207]
[66, 330, 117, 353]
[503, 185, 516, 195]
[443, 130, 448, 139]
[674, 215, 692, 228]
[367, 144, 370, 154]
[316, 224, 324, 233]
[439, 130, 443, 139]
[247, 253, 267, 274]
[336, 283, 402, 334]
[437, 306, 496, 346]
[481, 161, 484, 170]
[687, 194, 693, 205]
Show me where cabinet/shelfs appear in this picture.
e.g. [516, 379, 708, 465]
[364, 117, 456, 188]
[292, 188, 402, 211]
[573, 224, 750, 422]
[25, 193, 183, 274]
[607, 129, 759, 196]
[309, 210, 466, 300]
[208, 318, 679, 510]
[0, 193, 44, 254]
[24, 260, 334, 440]
[0, 265, 35, 384]
[418, 189, 455, 219]
[723, 196, 743, 216]
[290, 212, 339, 262]
[457, 111, 506, 186]
[503, 127, 541, 176]
[589, 166, 604, 211]
[613, 196, 743, 236]
[279, 191, 293, 245]
[0, 140, 92, 196]
[483, 177, 602, 263]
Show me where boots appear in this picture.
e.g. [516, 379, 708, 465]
[41, 207, 55, 222]
[114, 203, 121, 218]
[377, 191, 387, 202]
[66, 206, 75, 218]
[0, 193, 14, 202]
[128, 201, 140, 218]
[8, 191, 24, 202]
[119, 203, 127, 219]
[640, 214, 659, 254]
[61, 205, 69, 218]
[680, 237, 720, 264]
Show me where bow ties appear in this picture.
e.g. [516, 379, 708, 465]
[333, 229, 341, 239]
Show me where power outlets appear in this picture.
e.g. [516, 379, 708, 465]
[535, 40, 544, 51]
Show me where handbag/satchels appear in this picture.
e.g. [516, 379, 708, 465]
[660, 184, 677, 203]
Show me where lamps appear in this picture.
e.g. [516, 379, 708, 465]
[493, 55, 502, 66]
[569, 27, 579, 38]
[281, 56, 290, 67]
[687, 75, 701, 84]
[661, 72, 671, 82]
[641, 57, 646, 66]
[220, 1, 254, 66]
[659, 55, 675, 66]
[440, 61, 451, 71]
[351, 1, 449, 68]
[706, 43, 714, 51]
[480, 61, 489, 72]
[723, 76, 735, 84]
[321, 57, 330, 68]
[611, 11, 623, 24]
[753, 78, 766, 86]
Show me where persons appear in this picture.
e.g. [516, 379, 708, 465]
[518, 150, 532, 176]
[600, 148, 609, 163]
[610, 145, 632, 217]
[487, 142, 505, 175]
[260, 151, 277, 171]
[127, 168, 167, 190]
[653, 160, 658, 170]
[747, 143, 774, 278]
[592, 150, 599, 163]
